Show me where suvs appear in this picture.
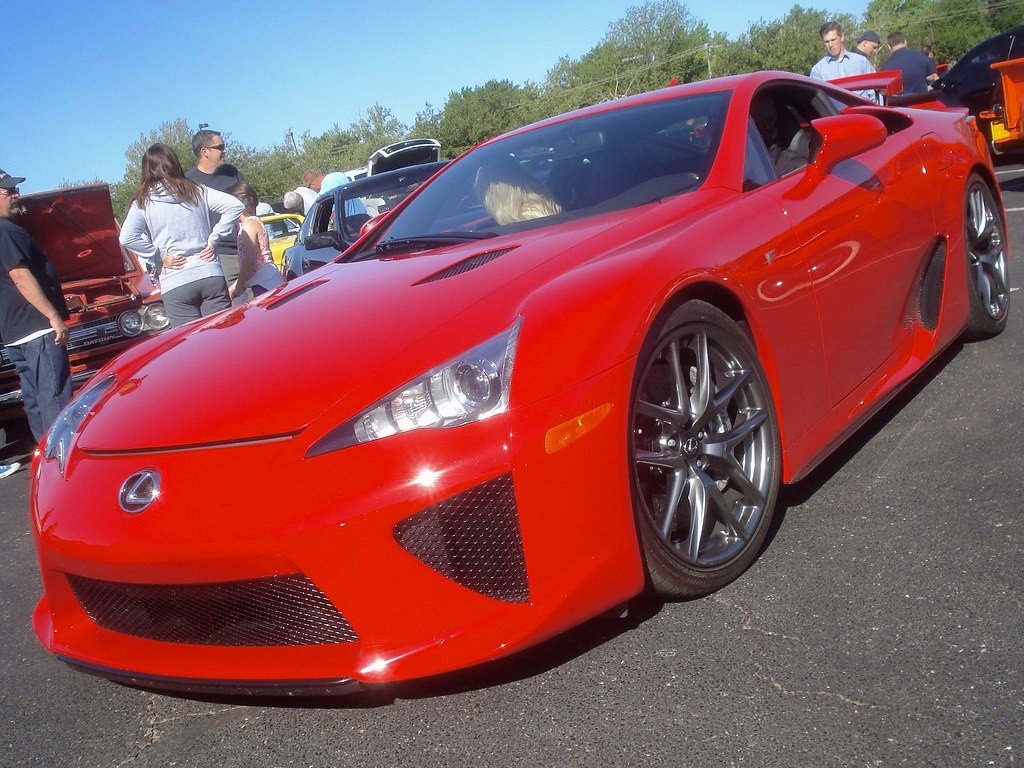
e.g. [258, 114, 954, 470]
[0, 182, 170, 417]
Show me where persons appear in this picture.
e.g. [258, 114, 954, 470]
[810, 21, 884, 112]
[474, 158, 565, 226]
[985, 48, 1001, 61]
[921, 45, 935, 61]
[0, 170, 73, 446]
[0, 461, 22, 480]
[119, 143, 246, 331]
[848, 30, 880, 59]
[256, 170, 372, 241]
[222, 179, 286, 308]
[880, 32, 940, 95]
[183, 129, 247, 196]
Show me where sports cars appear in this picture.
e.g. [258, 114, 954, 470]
[29, 69, 1010, 697]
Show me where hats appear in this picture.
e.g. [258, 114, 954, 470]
[855, 31, 880, 43]
[0, 170, 26, 187]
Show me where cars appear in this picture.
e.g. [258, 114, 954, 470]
[344, 139, 441, 217]
[280, 161, 498, 281]
[931, 24, 1024, 122]
[256, 213, 305, 272]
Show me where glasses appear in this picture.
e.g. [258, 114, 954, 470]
[204, 144, 225, 151]
[0, 187, 19, 196]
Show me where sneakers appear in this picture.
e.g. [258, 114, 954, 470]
[0, 462, 21, 479]
